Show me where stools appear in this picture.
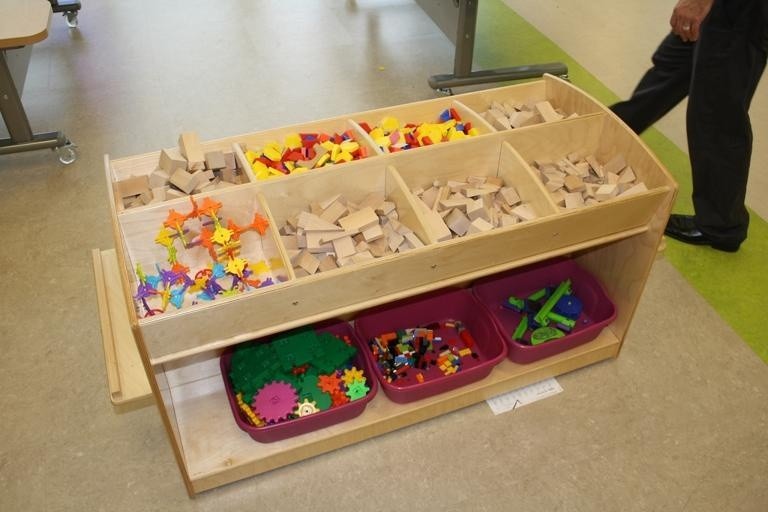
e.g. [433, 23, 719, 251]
[1, 0, 82, 156]
[427, 0, 567, 91]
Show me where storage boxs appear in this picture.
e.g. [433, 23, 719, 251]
[472, 257, 619, 365]
[355, 287, 508, 404]
[219, 317, 381, 443]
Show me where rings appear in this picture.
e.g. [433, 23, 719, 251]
[683, 25, 690, 31]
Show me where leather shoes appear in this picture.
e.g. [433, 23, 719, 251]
[663, 212, 743, 253]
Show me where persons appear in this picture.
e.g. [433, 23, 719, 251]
[608, 1, 767, 252]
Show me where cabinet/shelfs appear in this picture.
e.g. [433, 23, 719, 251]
[91, 70, 679, 499]
